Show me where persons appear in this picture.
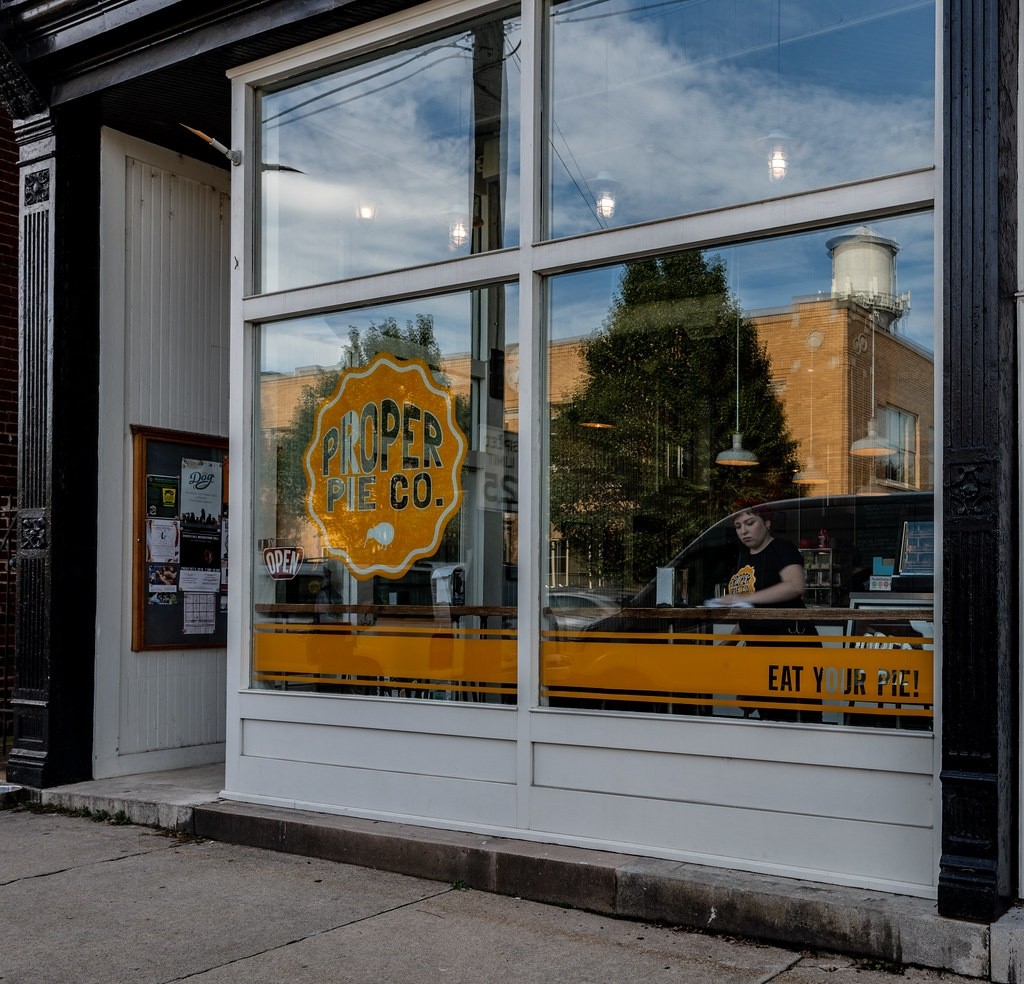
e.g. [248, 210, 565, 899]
[715, 501, 824, 723]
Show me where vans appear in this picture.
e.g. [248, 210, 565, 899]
[550, 490, 937, 738]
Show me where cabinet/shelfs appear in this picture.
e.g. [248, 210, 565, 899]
[797, 548, 832, 605]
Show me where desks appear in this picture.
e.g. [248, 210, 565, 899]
[542, 607, 934, 732]
[254, 604, 517, 705]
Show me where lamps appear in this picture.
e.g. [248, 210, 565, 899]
[588, 171, 623, 221]
[714, 246, 761, 466]
[792, 352, 827, 485]
[849, 307, 898, 457]
[759, 2, 792, 184]
[443, 202, 472, 249]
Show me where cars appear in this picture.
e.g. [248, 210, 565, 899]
[268, 558, 638, 707]
